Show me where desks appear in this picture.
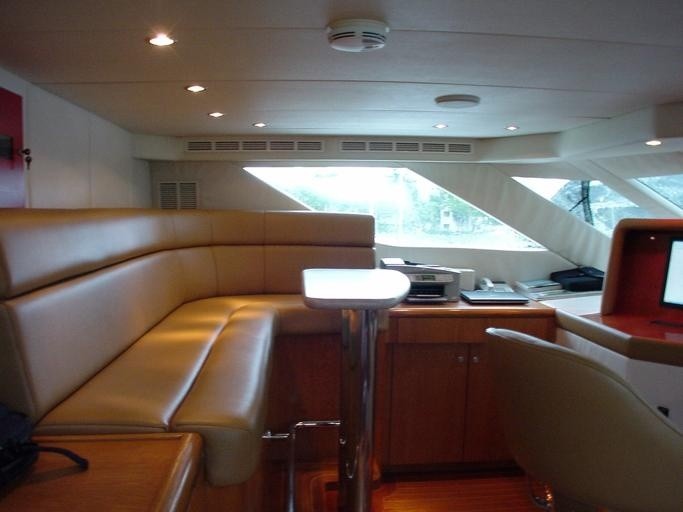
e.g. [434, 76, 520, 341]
[301, 269, 411, 511]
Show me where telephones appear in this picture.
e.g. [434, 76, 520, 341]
[478, 277, 513, 292]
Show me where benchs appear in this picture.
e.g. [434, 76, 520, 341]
[0, 206, 376, 485]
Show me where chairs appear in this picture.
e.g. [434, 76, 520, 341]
[484, 327, 681, 511]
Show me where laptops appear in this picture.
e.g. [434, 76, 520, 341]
[460, 290, 531, 306]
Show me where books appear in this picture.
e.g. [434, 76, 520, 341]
[515, 278, 569, 301]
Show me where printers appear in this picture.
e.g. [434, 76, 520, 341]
[380, 256, 461, 304]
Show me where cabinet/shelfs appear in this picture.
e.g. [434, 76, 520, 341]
[385, 295, 555, 478]
[0, 432, 262, 510]
